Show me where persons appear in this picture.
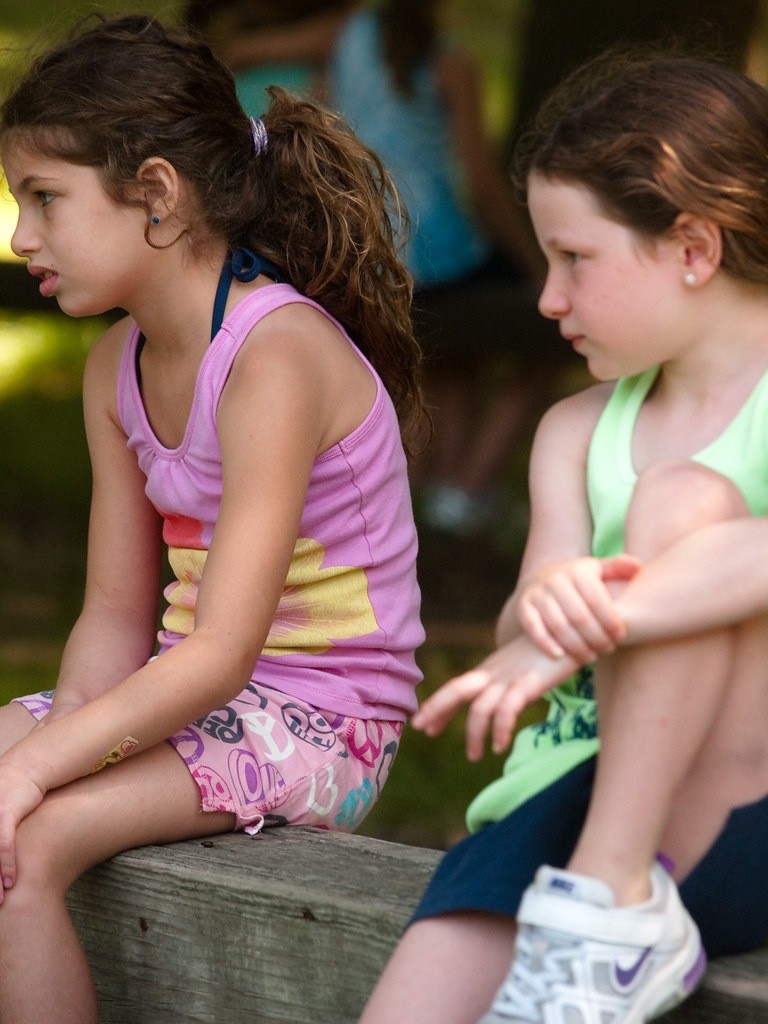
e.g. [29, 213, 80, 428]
[358, 54, 768, 1024]
[0, 13, 431, 1023]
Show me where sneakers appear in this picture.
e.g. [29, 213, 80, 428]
[480, 850, 704, 1022]
